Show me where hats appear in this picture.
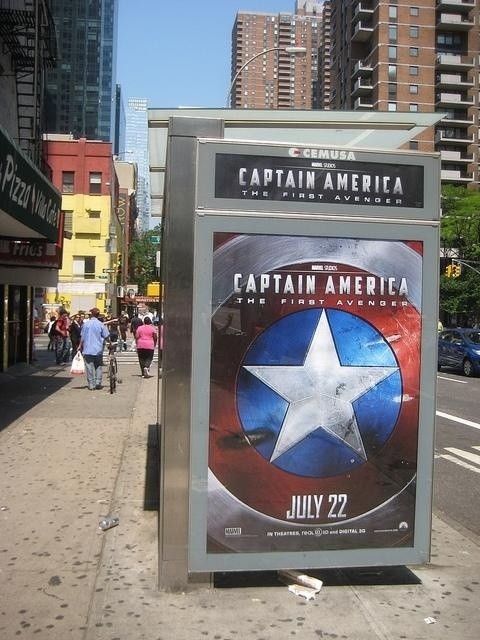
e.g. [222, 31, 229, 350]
[92, 308, 99, 316]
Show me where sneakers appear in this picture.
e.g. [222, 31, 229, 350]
[58, 362, 66, 366]
[143, 367, 149, 377]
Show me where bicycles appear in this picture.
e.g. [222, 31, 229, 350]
[104, 341, 126, 394]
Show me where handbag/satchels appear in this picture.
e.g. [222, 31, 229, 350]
[50, 320, 59, 336]
[44, 323, 49, 333]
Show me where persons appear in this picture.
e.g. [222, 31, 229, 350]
[40, 309, 159, 379]
[76, 307, 113, 390]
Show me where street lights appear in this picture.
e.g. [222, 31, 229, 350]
[224, 46, 307, 108]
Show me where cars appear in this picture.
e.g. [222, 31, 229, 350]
[438, 327, 480, 378]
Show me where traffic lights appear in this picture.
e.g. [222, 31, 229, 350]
[455, 266, 462, 278]
[445, 265, 452, 277]
[116, 252, 122, 274]
[452, 265, 457, 274]
